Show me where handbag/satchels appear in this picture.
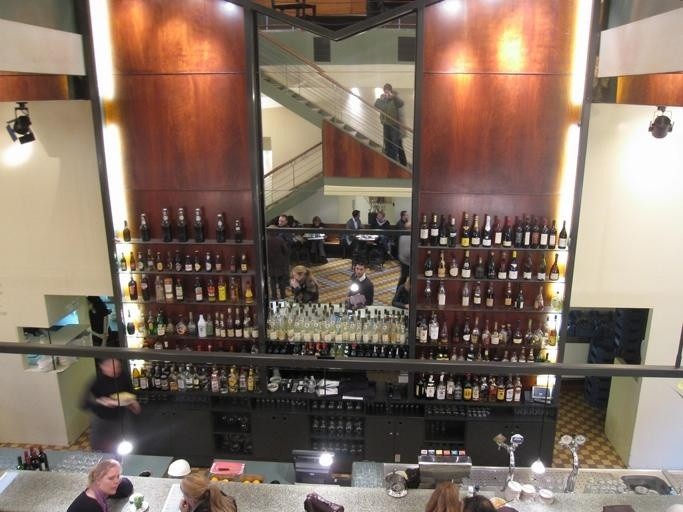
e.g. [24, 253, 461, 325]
[304, 492, 344, 512]
[344, 294, 366, 310]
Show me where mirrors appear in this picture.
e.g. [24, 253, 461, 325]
[229, 0, 444, 403]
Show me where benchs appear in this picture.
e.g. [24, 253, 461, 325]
[301, 224, 411, 258]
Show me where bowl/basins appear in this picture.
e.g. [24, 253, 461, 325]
[266, 375, 281, 392]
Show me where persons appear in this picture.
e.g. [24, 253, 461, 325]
[345, 210, 366, 258]
[463, 495, 515, 512]
[346, 261, 374, 307]
[276, 213, 308, 262]
[289, 265, 319, 305]
[83, 355, 145, 424]
[309, 215, 327, 264]
[392, 275, 411, 309]
[370, 212, 393, 259]
[394, 222, 411, 292]
[84, 295, 120, 376]
[177, 473, 238, 512]
[425, 481, 463, 512]
[375, 83, 404, 162]
[266, 225, 288, 300]
[64, 459, 122, 511]
[394, 211, 411, 259]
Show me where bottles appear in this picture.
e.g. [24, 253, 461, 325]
[140, 213, 150, 241]
[122, 307, 260, 354]
[415, 370, 522, 402]
[550, 290, 560, 312]
[461, 250, 470, 278]
[116, 245, 254, 305]
[423, 250, 433, 277]
[11, 444, 49, 471]
[461, 282, 469, 306]
[267, 303, 410, 360]
[419, 210, 570, 251]
[533, 285, 543, 311]
[485, 281, 494, 308]
[210, 397, 488, 459]
[281, 373, 317, 393]
[123, 221, 130, 242]
[215, 213, 226, 242]
[161, 208, 171, 241]
[423, 279, 432, 305]
[521, 253, 532, 279]
[497, 252, 506, 279]
[193, 208, 205, 243]
[436, 282, 446, 305]
[449, 252, 458, 276]
[549, 254, 559, 280]
[514, 286, 523, 310]
[233, 217, 242, 243]
[487, 252, 496, 279]
[472, 282, 481, 306]
[131, 358, 259, 392]
[474, 255, 484, 278]
[503, 281, 512, 305]
[177, 207, 186, 241]
[509, 250, 517, 279]
[502, 479, 555, 504]
[537, 254, 545, 280]
[437, 250, 445, 277]
[413, 312, 557, 364]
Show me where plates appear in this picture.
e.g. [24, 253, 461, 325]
[128, 492, 148, 511]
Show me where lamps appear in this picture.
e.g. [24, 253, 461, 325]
[648, 106, 676, 138]
[6, 101, 36, 144]
